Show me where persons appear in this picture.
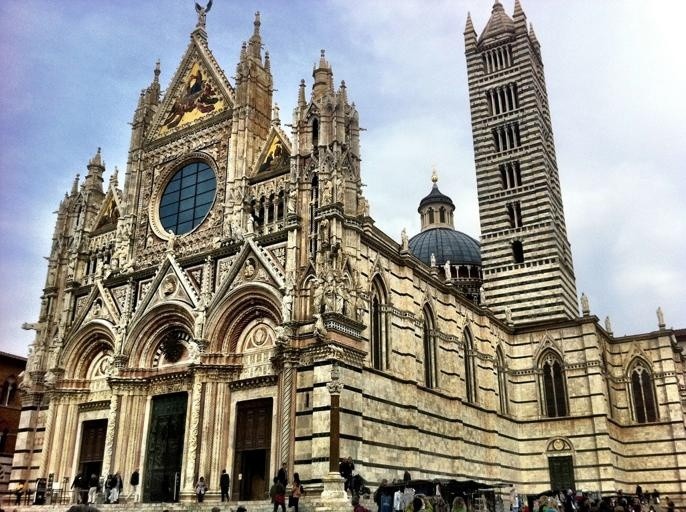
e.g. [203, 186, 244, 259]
[195, 476, 208, 501]
[220, 469, 230, 501]
[269, 476, 287, 512]
[70, 468, 125, 505]
[340, 456, 367, 511]
[372, 478, 674, 512]
[291, 472, 302, 511]
[278, 462, 288, 489]
[124, 468, 140, 502]
[14, 480, 24, 506]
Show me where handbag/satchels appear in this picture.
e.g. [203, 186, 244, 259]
[289, 495, 293, 507]
[274, 494, 285, 505]
